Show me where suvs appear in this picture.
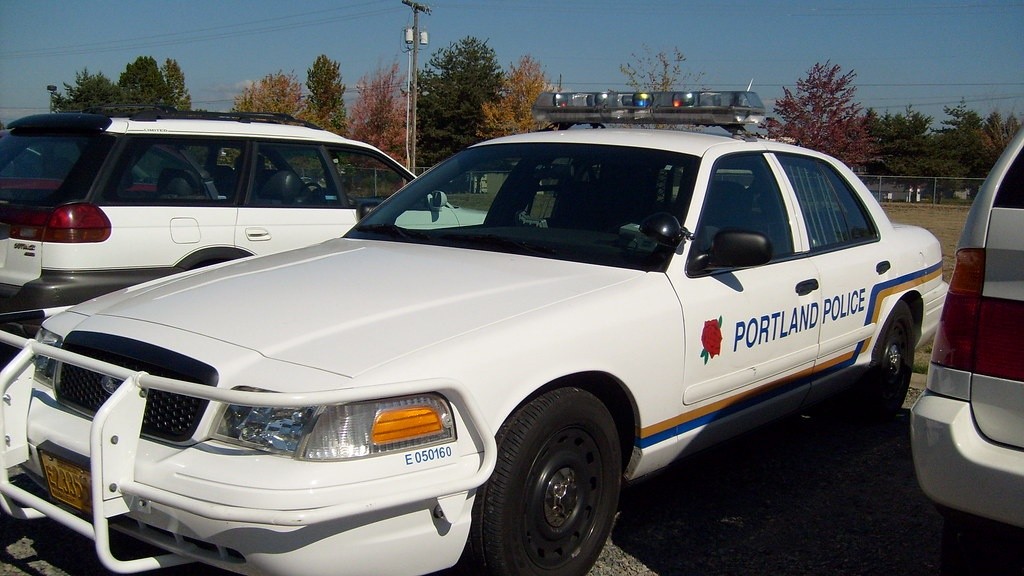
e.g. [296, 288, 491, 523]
[912, 123, 1024, 551]
[0, 110, 490, 375]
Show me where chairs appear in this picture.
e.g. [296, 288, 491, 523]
[699, 181, 770, 250]
[516, 165, 584, 229]
[256, 168, 303, 204]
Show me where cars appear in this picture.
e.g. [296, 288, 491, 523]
[1, 92, 951, 575]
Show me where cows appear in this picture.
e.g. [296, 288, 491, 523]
[953, 188, 971, 199]
